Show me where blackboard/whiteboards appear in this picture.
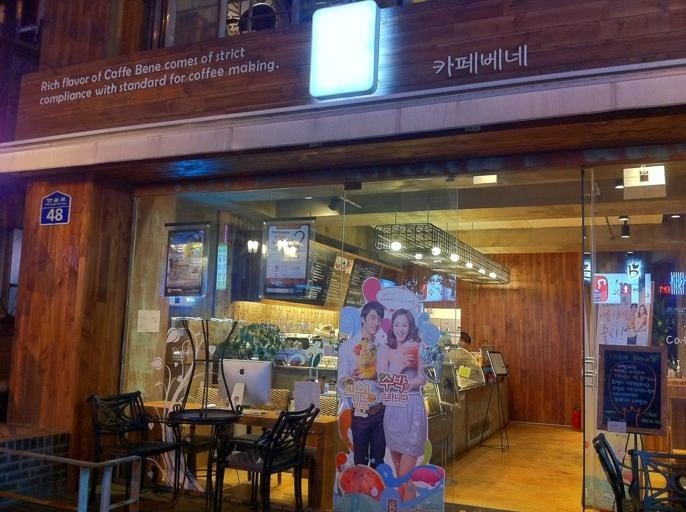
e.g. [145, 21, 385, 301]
[343, 258, 382, 309]
[263, 240, 338, 306]
[596, 344, 667, 436]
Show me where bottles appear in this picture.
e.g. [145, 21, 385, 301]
[330, 330, 336, 345]
[324, 384, 328, 394]
[287, 400, 294, 416]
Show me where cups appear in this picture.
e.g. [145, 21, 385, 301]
[668, 367, 677, 379]
[356, 349, 378, 380]
[401, 341, 420, 368]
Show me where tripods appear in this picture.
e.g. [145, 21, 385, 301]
[478, 377, 510, 453]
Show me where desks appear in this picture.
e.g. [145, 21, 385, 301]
[167, 317, 244, 510]
[140, 400, 340, 511]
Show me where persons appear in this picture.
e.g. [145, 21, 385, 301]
[377, 308, 427, 480]
[456, 330, 471, 350]
[337, 301, 388, 471]
[624, 304, 637, 345]
[637, 304, 649, 346]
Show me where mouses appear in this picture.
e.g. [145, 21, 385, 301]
[207, 404, 217, 408]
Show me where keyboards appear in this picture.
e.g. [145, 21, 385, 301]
[243, 409, 267, 414]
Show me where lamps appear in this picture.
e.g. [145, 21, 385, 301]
[613, 179, 626, 190]
[372, 195, 512, 286]
[619, 215, 629, 221]
[620, 220, 632, 239]
[327, 195, 342, 210]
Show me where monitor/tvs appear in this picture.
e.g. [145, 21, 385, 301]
[217, 358, 272, 413]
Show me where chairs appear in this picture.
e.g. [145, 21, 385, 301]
[321, 394, 338, 417]
[627, 445, 686, 511]
[591, 431, 646, 511]
[90, 390, 182, 507]
[218, 403, 318, 511]
